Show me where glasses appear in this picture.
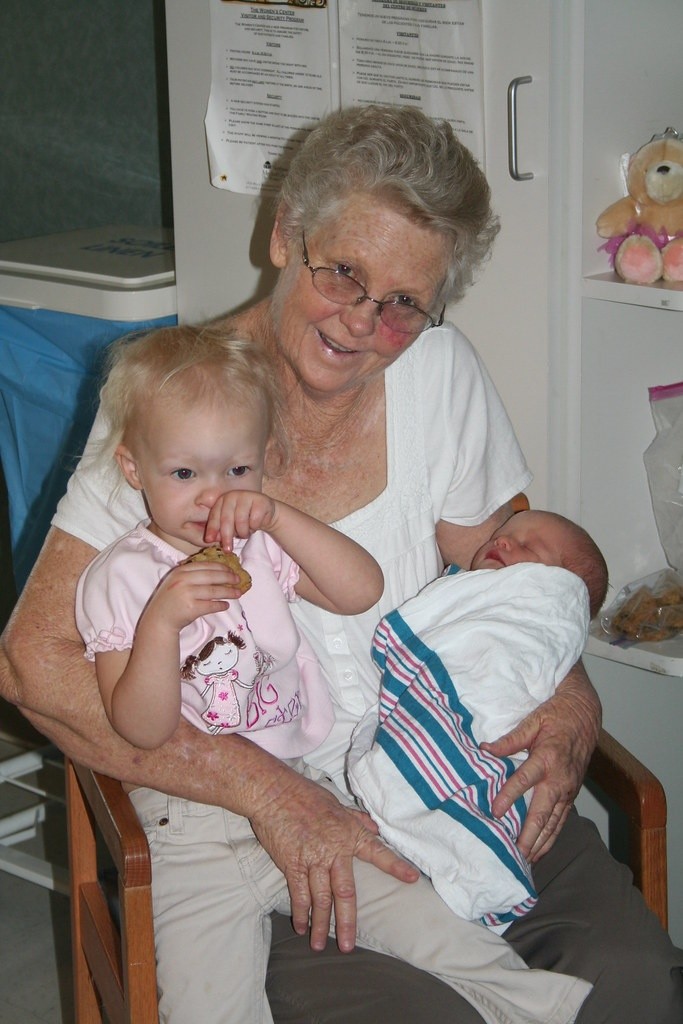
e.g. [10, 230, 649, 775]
[300, 224, 448, 335]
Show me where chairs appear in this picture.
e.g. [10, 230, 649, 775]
[65, 743, 670, 1023]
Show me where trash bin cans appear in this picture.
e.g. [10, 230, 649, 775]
[0, 224, 179, 599]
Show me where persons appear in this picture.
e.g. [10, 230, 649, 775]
[347, 511, 609, 937]
[0, 104, 683, 1024]
[75, 327, 593, 1024]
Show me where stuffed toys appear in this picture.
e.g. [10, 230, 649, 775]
[596, 127, 683, 284]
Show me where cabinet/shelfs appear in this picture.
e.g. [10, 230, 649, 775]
[573, 2, 683, 949]
